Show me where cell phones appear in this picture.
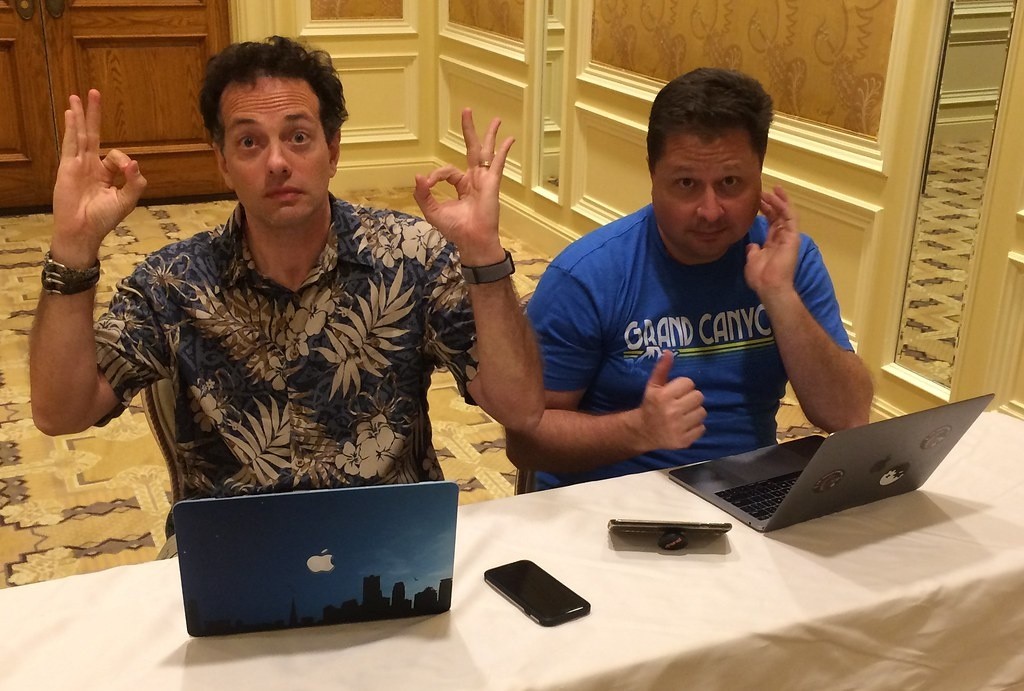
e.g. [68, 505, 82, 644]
[484, 560, 590, 626]
[607, 519, 732, 537]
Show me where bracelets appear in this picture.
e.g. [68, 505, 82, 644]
[41, 250, 100, 295]
[460, 248, 515, 284]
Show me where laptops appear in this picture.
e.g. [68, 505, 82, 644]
[669, 392, 996, 532]
[170, 481, 460, 638]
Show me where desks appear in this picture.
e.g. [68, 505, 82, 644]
[0, 411, 1024, 691]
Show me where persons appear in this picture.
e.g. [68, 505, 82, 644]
[505, 68, 875, 493]
[29, 35, 547, 562]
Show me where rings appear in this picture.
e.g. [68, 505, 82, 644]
[479, 161, 492, 167]
